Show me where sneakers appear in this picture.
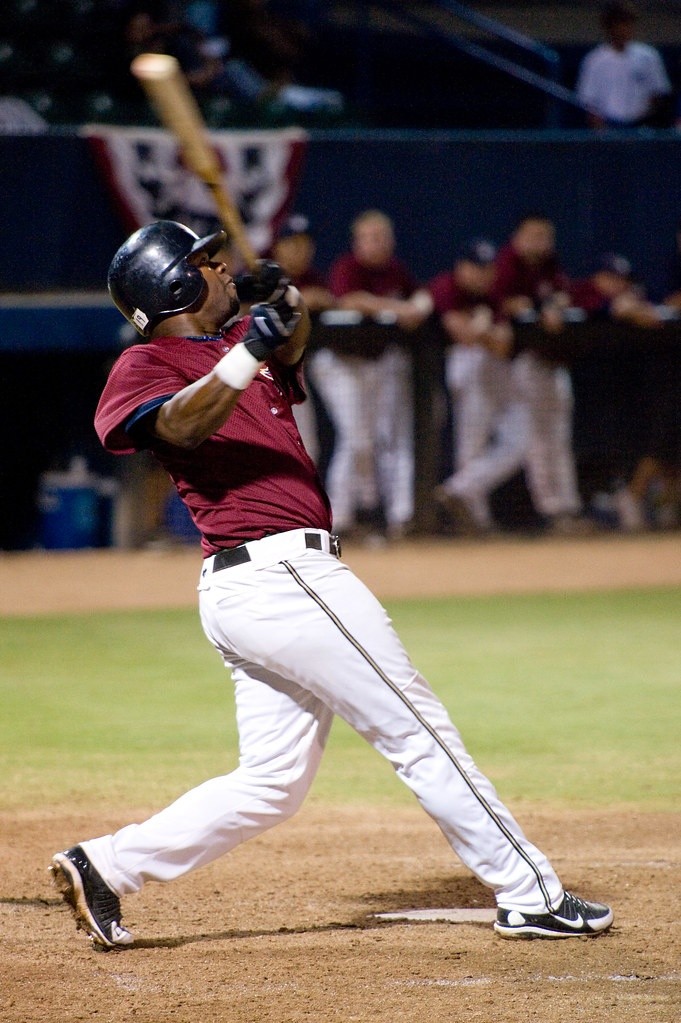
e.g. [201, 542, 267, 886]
[47, 845, 134, 948]
[493, 892, 615, 940]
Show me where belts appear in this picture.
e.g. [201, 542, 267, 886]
[213, 533, 342, 573]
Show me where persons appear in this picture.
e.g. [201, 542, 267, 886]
[46, 220, 613, 948]
[0, 0, 344, 133]
[575, 1, 675, 130]
[268, 211, 681, 537]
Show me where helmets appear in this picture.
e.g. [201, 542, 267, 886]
[107, 220, 227, 336]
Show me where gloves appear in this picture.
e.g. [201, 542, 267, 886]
[242, 304, 301, 362]
[234, 258, 292, 309]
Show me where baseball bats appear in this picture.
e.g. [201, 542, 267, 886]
[129, 52, 258, 274]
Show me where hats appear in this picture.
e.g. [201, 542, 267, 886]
[593, 250, 635, 282]
[462, 237, 498, 268]
[273, 214, 318, 243]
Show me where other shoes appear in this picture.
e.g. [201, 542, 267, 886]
[544, 517, 594, 536]
[432, 471, 497, 534]
[616, 488, 641, 531]
